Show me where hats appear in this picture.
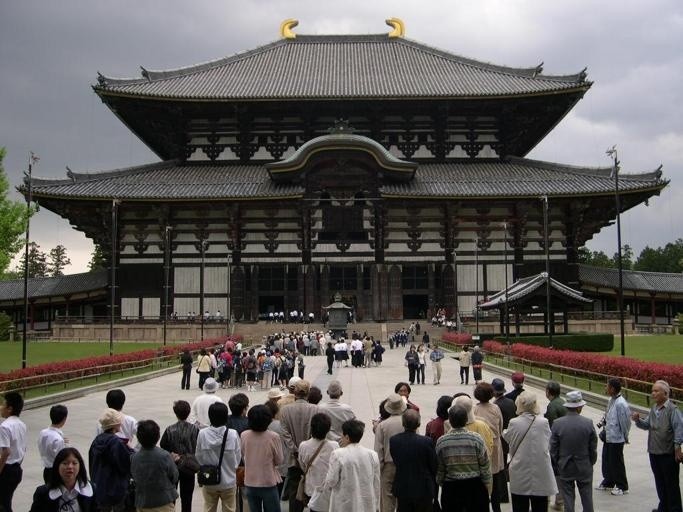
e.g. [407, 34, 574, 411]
[294, 380, 309, 395]
[202, 377, 219, 392]
[562, 390, 586, 408]
[267, 387, 284, 398]
[447, 395, 475, 425]
[512, 372, 524, 383]
[514, 390, 540, 415]
[98, 408, 122, 430]
[490, 378, 505, 394]
[287, 376, 302, 389]
[383, 393, 407, 415]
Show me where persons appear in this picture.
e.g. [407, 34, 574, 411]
[417, 344, 427, 384]
[37, 404, 71, 484]
[630, 378, 683, 512]
[430, 345, 443, 386]
[458, 344, 471, 386]
[86, 372, 600, 512]
[29, 446, 101, 512]
[594, 377, 632, 496]
[430, 305, 459, 332]
[405, 344, 420, 386]
[168, 308, 221, 325]
[181, 329, 386, 391]
[471, 345, 485, 385]
[0, 390, 28, 512]
[388, 321, 431, 353]
[261, 308, 317, 325]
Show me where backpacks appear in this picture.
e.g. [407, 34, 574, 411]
[279, 356, 288, 372]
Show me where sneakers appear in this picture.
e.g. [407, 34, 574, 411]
[610, 487, 629, 495]
[594, 484, 612, 490]
[550, 504, 562, 511]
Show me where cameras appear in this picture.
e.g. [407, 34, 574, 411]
[597, 418, 606, 428]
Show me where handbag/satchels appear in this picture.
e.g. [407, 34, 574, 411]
[295, 474, 308, 501]
[235, 466, 244, 487]
[177, 454, 200, 477]
[196, 369, 199, 373]
[197, 465, 220, 486]
